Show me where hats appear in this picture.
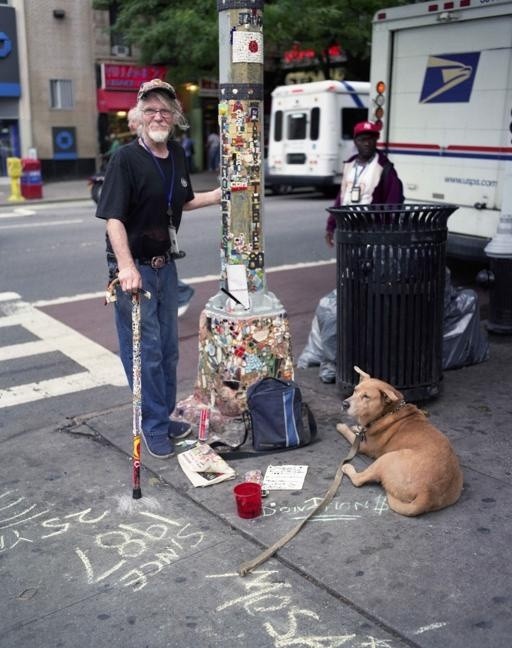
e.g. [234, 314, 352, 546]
[137, 79, 177, 99]
[353, 121, 379, 138]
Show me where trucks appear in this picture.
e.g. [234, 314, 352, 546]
[264, 77, 369, 198]
[367, 0, 511, 264]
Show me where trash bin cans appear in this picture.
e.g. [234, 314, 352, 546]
[325, 203, 459, 404]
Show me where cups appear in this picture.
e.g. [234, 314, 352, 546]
[234, 483, 262, 518]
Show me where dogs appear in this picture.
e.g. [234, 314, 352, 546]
[336, 363, 464, 518]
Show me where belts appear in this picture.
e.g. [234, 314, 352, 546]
[107, 251, 172, 269]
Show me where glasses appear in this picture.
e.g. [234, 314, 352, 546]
[141, 109, 172, 115]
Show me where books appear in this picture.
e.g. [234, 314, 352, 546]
[176, 442, 238, 488]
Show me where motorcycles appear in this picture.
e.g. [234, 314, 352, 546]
[86, 154, 111, 204]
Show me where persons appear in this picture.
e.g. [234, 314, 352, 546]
[98, 128, 222, 173]
[325, 120, 404, 254]
[95, 77, 222, 459]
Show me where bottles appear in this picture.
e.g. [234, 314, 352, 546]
[198, 407, 210, 441]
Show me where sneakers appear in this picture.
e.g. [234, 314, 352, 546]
[141, 421, 192, 459]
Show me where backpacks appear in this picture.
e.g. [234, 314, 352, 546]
[247, 377, 309, 451]
